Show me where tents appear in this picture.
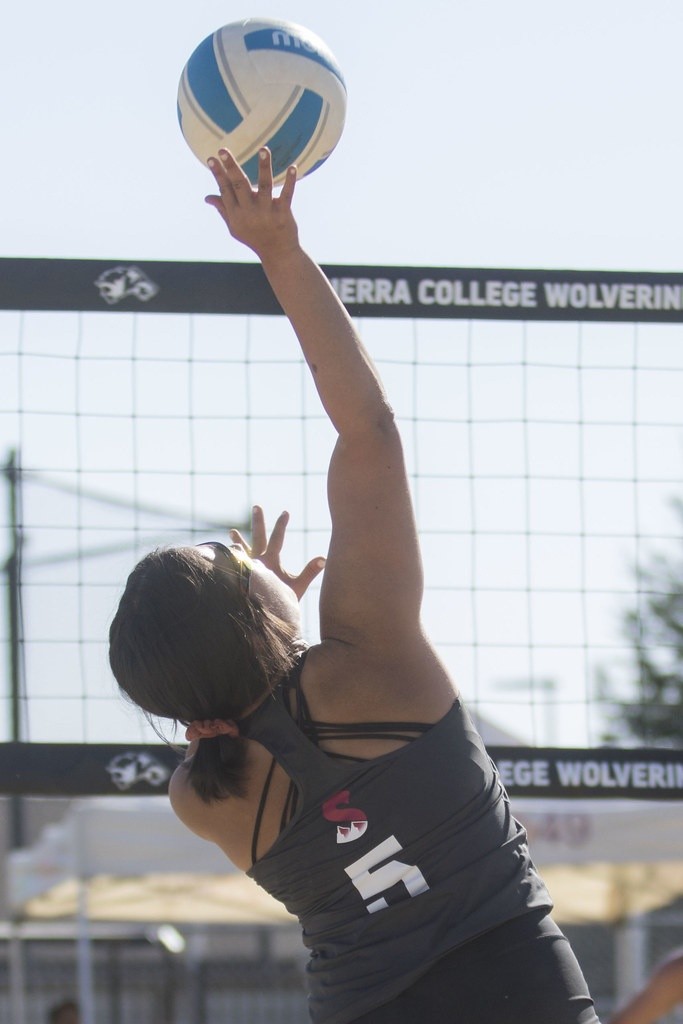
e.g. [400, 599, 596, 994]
[0, 686, 683, 1024]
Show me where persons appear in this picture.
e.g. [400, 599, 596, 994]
[48, 1001, 81, 1024]
[110, 144, 601, 1024]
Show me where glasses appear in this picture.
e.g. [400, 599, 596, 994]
[194, 541, 254, 610]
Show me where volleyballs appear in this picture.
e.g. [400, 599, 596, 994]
[175, 14, 351, 188]
